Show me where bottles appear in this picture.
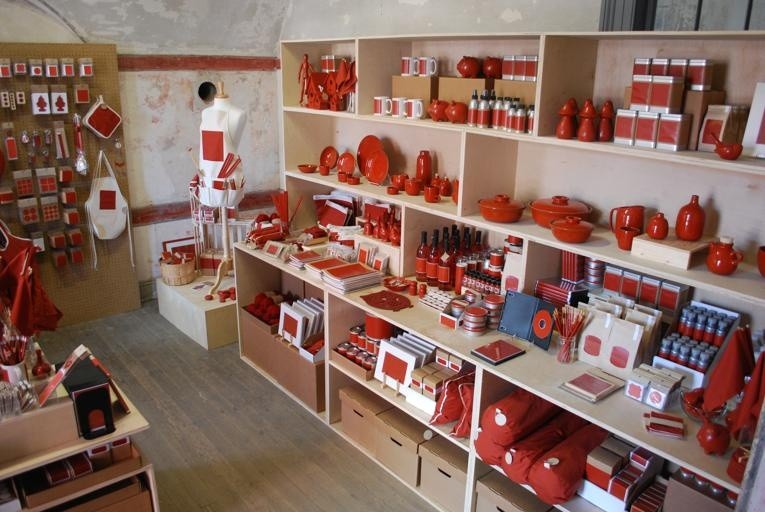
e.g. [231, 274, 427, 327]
[729, 318, 729, 319]
[450, 236, 463, 264]
[526, 104, 535, 135]
[516, 104, 527, 134]
[467, 90, 479, 128]
[373, 341, 380, 359]
[667, 332, 698, 348]
[659, 338, 673, 360]
[454, 256, 468, 296]
[442, 232, 454, 256]
[366, 337, 374, 355]
[713, 321, 729, 348]
[693, 315, 707, 341]
[486, 293, 506, 330]
[450, 299, 469, 319]
[507, 105, 515, 133]
[715, 313, 736, 324]
[349, 327, 361, 345]
[678, 309, 691, 334]
[462, 269, 501, 295]
[477, 90, 489, 129]
[451, 229, 460, 253]
[461, 234, 473, 258]
[357, 331, 367, 351]
[676, 345, 690, 367]
[466, 253, 478, 272]
[355, 351, 368, 367]
[685, 304, 716, 317]
[689, 348, 701, 370]
[682, 312, 696, 340]
[415, 231, 430, 283]
[440, 226, 449, 247]
[437, 238, 454, 291]
[500, 97, 511, 132]
[463, 306, 488, 338]
[473, 230, 485, 255]
[513, 97, 520, 110]
[696, 340, 719, 356]
[336, 342, 351, 357]
[493, 97, 503, 132]
[429, 229, 441, 250]
[465, 288, 482, 303]
[461, 226, 470, 254]
[489, 93, 496, 128]
[696, 352, 709, 373]
[346, 346, 360, 362]
[702, 318, 718, 344]
[362, 356, 377, 371]
[426, 237, 441, 287]
[449, 224, 457, 244]
[667, 342, 682, 363]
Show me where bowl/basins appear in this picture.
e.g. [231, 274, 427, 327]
[297, 164, 317, 173]
[714, 144, 743, 160]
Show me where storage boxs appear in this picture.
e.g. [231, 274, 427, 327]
[240, 295, 325, 414]
[663, 469, 738, 512]
[475, 469, 561, 512]
[339, 390, 396, 454]
[370, 409, 439, 487]
[418, 435, 467, 512]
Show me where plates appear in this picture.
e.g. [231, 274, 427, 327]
[357, 135, 384, 176]
[365, 150, 389, 184]
[384, 276, 412, 291]
[337, 152, 356, 176]
[320, 146, 339, 169]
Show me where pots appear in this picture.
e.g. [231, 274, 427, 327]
[528, 196, 592, 230]
[549, 216, 594, 244]
[477, 194, 526, 223]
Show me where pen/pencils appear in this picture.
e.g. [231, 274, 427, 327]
[0, 334, 31, 384]
[550, 305, 589, 363]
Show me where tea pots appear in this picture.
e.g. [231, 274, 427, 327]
[610, 206, 646, 239]
[707, 236, 743, 275]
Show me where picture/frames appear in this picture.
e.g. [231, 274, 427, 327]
[72, 383, 115, 440]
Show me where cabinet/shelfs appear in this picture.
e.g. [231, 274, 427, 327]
[0, 341, 160, 510]
[234, 29, 765, 512]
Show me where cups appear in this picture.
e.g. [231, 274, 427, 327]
[405, 180, 421, 195]
[423, 185, 441, 203]
[388, 186, 399, 195]
[0, 359, 30, 385]
[419, 57, 437, 76]
[407, 99, 423, 119]
[373, 96, 391, 115]
[391, 174, 410, 191]
[557, 337, 575, 365]
[338, 172, 348, 182]
[348, 176, 360, 184]
[618, 227, 640, 251]
[391, 97, 408, 117]
[757, 245, 765, 277]
[319, 165, 330, 176]
[401, 57, 419, 76]
